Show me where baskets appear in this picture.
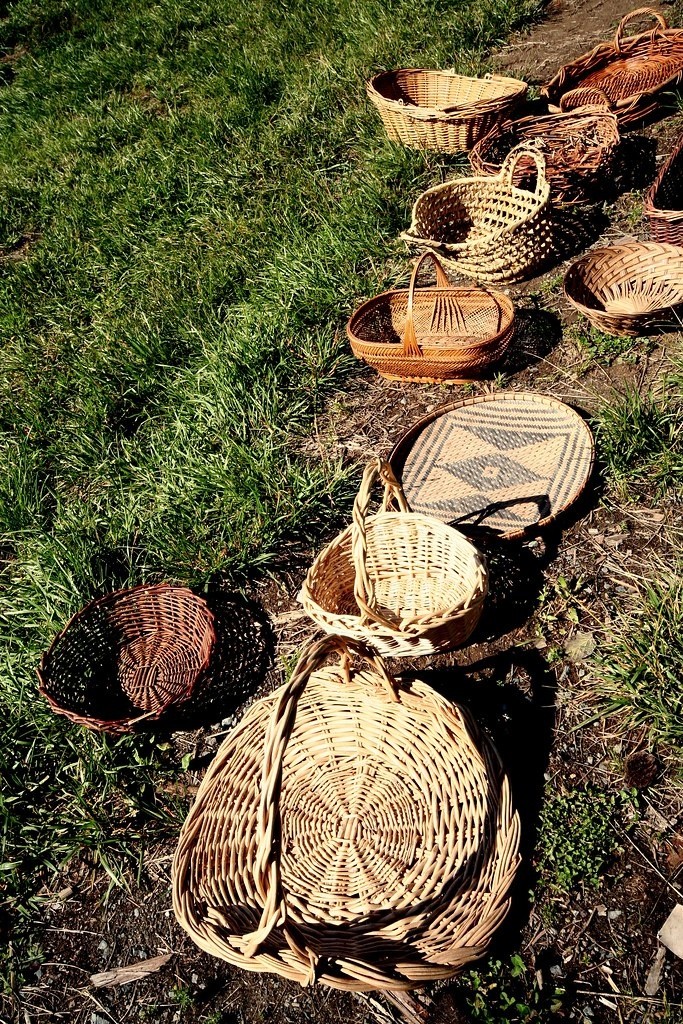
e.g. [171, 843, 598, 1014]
[564, 241, 683, 336]
[540, 8, 683, 133]
[32, 582, 216, 730]
[168, 631, 523, 992]
[384, 392, 595, 544]
[365, 67, 529, 156]
[299, 451, 489, 657]
[466, 110, 621, 219]
[399, 143, 555, 287]
[347, 250, 517, 385]
[642, 136, 683, 246]
